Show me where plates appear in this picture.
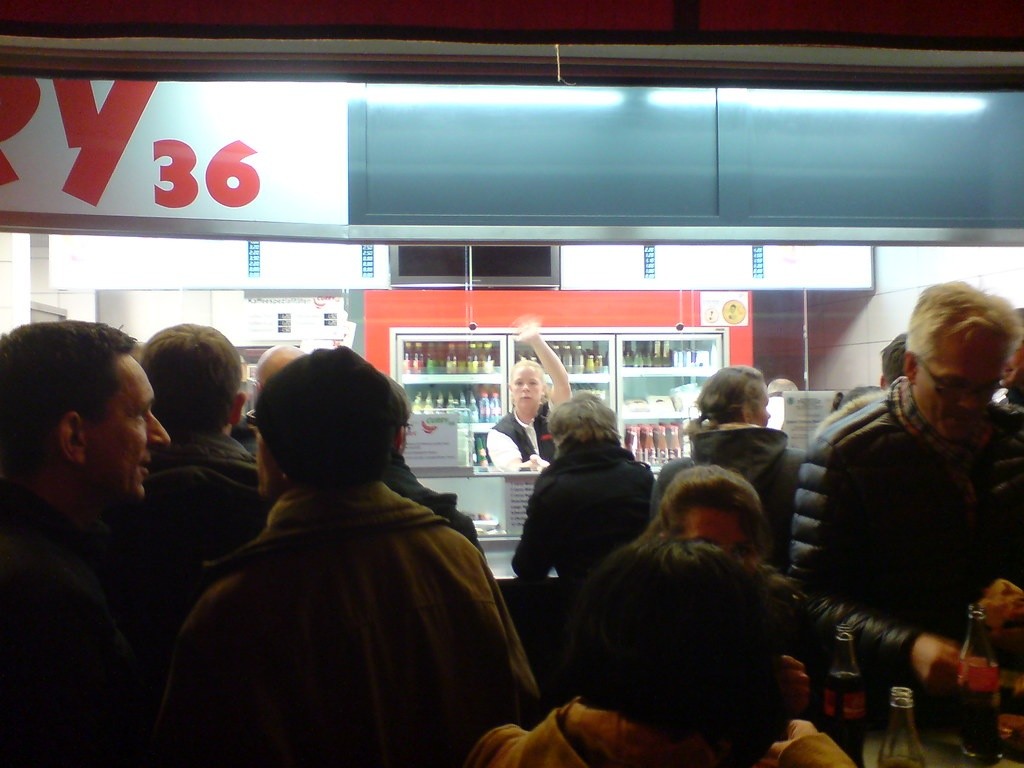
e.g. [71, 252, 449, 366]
[474, 520, 498, 530]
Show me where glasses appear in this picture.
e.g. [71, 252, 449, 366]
[919, 361, 1000, 399]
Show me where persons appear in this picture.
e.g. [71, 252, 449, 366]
[0, 322, 478, 768]
[465, 535, 858, 768]
[487, 315, 572, 470]
[152, 346, 540, 768]
[512, 281, 1024, 732]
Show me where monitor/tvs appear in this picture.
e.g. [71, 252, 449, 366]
[389, 244, 561, 290]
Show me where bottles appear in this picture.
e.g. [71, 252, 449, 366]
[625, 422, 682, 467]
[878, 687, 926, 768]
[475, 435, 490, 467]
[403, 343, 495, 374]
[820, 622, 868, 768]
[956, 604, 1004, 764]
[624, 350, 674, 367]
[409, 390, 502, 423]
[516, 345, 603, 374]
